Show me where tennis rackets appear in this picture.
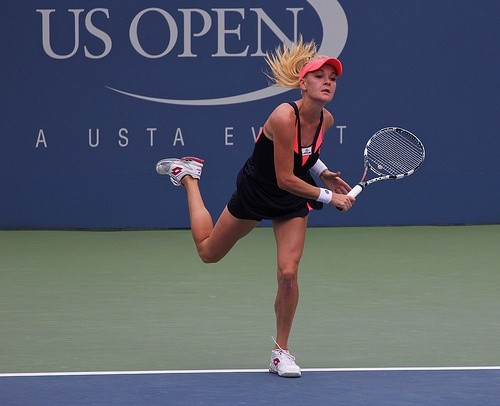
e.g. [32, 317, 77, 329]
[335, 127, 426, 212]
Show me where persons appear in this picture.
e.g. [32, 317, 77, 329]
[151, 39, 353, 379]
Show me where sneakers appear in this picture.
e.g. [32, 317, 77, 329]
[156, 156, 204, 186]
[269, 336, 301, 377]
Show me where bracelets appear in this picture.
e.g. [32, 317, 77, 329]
[309, 157, 330, 179]
[316, 188, 333, 205]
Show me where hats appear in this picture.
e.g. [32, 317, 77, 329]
[300, 57, 342, 81]
